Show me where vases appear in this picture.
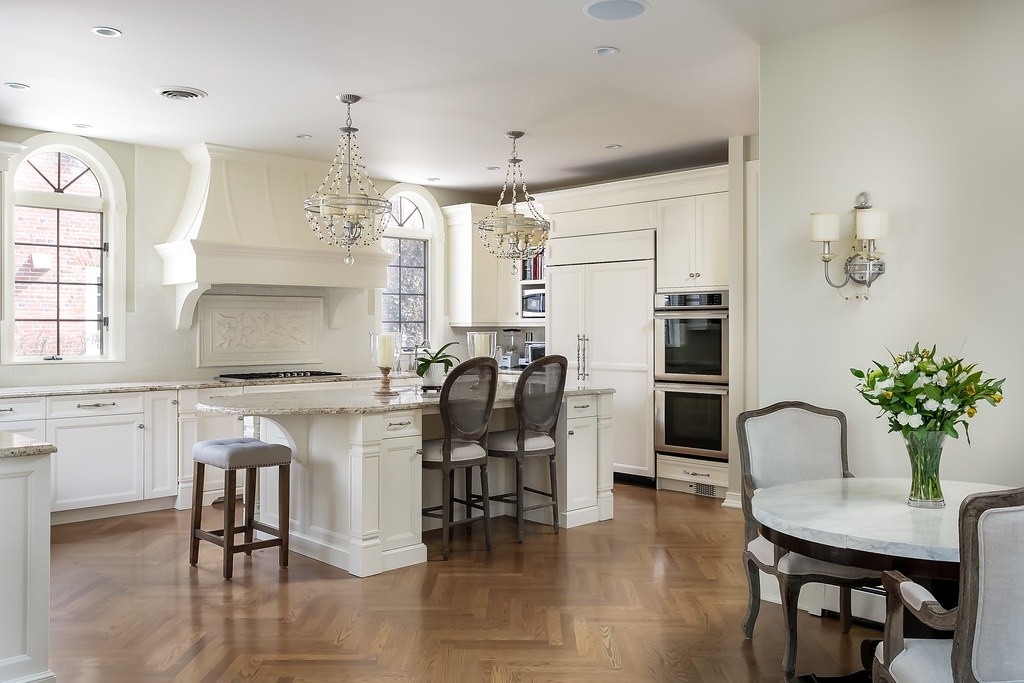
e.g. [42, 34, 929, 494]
[899, 425, 956, 510]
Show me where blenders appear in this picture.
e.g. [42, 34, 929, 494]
[499, 329, 521, 367]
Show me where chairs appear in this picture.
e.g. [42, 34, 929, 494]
[464, 346, 573, 547]
[423, 356, 498, 560]
[733, 398, 885, 683]
[870, 484, 1024, 683]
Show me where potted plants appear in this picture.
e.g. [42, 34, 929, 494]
[415, 336, 461, 394]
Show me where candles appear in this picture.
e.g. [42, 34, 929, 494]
[375, 332, 396, 367]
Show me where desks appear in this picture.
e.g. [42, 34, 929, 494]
[193, 377, 623, 578]
[749, 486, 1024, 681]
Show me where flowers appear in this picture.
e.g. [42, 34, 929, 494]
[848, 341, 1006, 499]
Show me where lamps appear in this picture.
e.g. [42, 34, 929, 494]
[476, 128, 553, 278]
[809, 191, 888, 301]
[304, 89, 393, 268]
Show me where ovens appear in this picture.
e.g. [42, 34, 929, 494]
[654, 292, 729, 461]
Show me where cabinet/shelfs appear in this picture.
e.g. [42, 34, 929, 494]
[172, 387, 244, 510]
[445, 165, 731, 499]
[0, 390, 180, 683]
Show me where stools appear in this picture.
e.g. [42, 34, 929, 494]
[184, 433, 295, 585]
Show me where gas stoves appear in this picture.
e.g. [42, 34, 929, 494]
[220, 371, 342, 379]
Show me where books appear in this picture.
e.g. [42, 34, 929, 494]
[523, 252, 543, 279]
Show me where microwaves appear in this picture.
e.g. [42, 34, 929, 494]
[522, 289, 546, 318]
[529, 344, 545, 364]
